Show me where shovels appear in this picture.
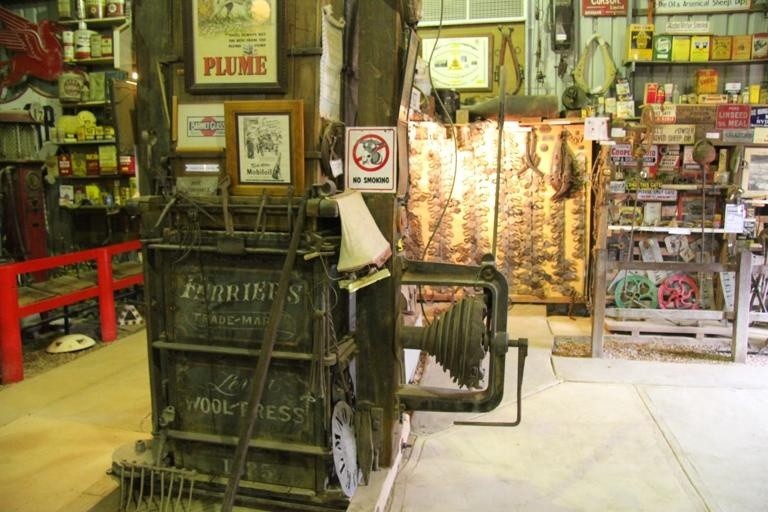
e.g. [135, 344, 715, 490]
[215, 175, 246, 255]
[693, 138, 716, 264]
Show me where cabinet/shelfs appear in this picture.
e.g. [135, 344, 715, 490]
[397, 119, 589, 304]
[591, 0, 768, 363]
[54, 0, 146, 210]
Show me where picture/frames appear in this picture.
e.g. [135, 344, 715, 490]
[224, 101, 304, 195]
[183, 1, 287, 96]
[420, 35, 490, 90]
[173, 98, 226, 154]
[344, 126, 399, 193]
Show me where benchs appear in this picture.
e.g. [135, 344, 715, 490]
[2, 241, 144, 389]
